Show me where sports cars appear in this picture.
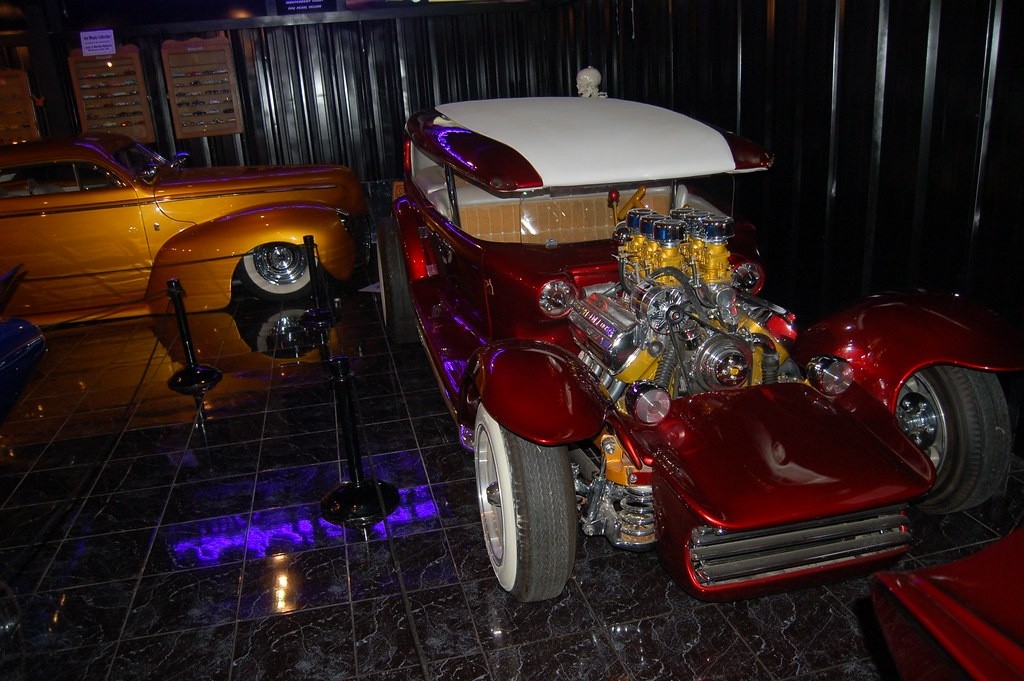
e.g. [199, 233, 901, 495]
[0, 132, 373, 327]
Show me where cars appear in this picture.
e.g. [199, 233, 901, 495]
[390, 93, 1024, 606]
[864, 528, 1023, 681]
